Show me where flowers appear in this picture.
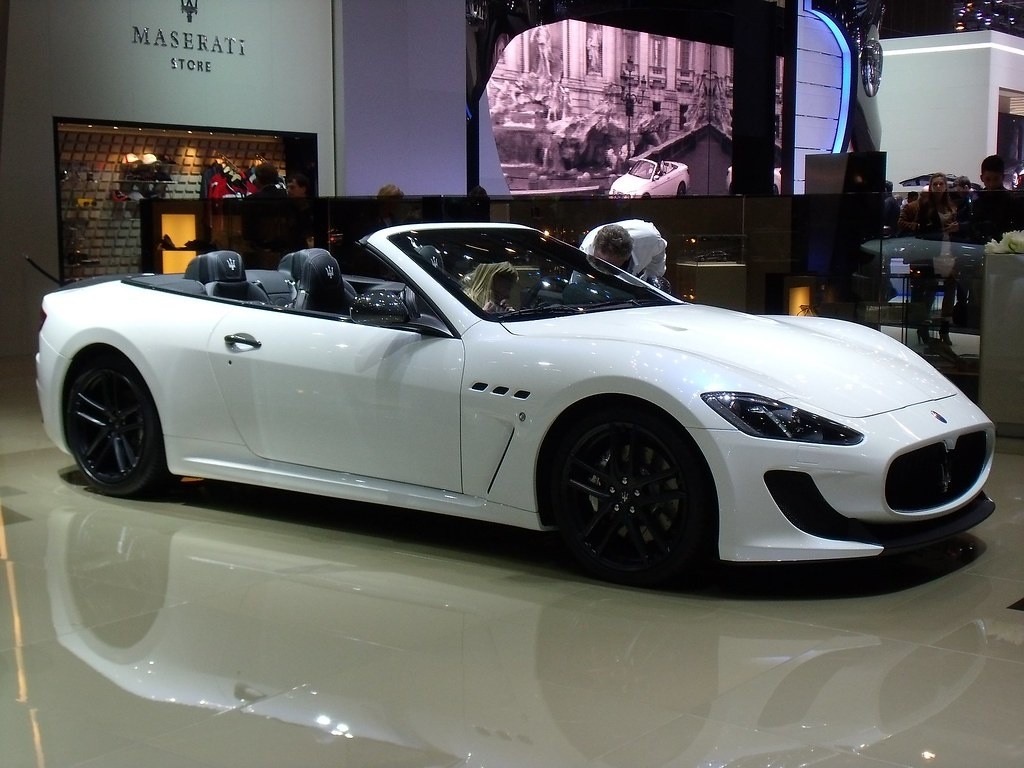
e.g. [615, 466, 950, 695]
[984, 230, 1024, 255]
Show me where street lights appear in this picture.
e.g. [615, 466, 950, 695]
[623, 59, 637, 169]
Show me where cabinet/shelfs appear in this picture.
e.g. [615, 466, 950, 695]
[111, 162, 179, 203]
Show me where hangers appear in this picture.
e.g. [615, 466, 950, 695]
[215, 157, 257, 184]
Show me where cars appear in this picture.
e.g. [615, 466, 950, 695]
[607, 158, 691, 199]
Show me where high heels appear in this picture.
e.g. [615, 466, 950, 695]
[917, 325, 930, 344]
[939, 326, 952, 345]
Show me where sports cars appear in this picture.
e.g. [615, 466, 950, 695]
[32, 222, 999, 588]
[848, 232, 989, 331]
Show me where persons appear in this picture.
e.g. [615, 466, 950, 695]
[650, 163, 663, 178]
[883, 155, 1017, 345]
[241, 163, 315, 269]
[359, 183, 411, 237]
[460, 260, 520, 314]
[528, 22, 556, 80]
[569, 219, 668, 293]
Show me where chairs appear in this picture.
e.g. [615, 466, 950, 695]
[291, 248, 357, 297]
[402, 244, 447, 321]
[204, 250, 270, 303]
[295, 253, 355, 315]
